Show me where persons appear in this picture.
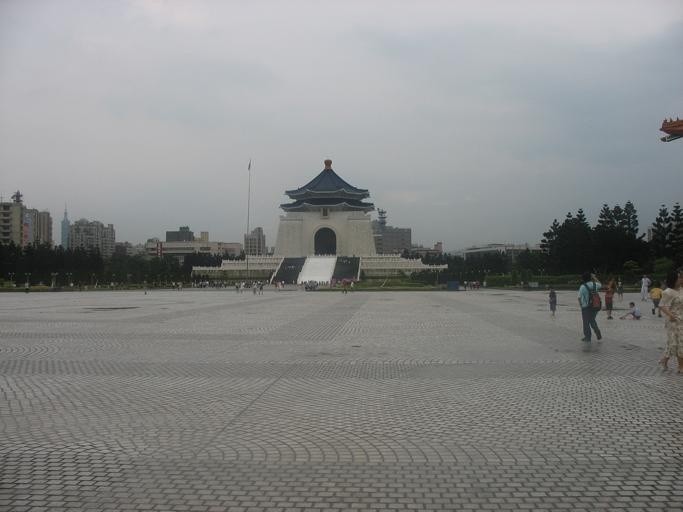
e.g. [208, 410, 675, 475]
[171, 278, 354, 295]
[462, 279, 480, 290]
[606, 270, 682, 372]
[548, 288, 556, 315]
[577, 272, 602, 341]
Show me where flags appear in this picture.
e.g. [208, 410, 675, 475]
[247, 162, 249, 170]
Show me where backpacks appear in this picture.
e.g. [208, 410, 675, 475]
[583, 282, 601, 310]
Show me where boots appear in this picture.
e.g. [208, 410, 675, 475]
[581, 337, 602, 341]
